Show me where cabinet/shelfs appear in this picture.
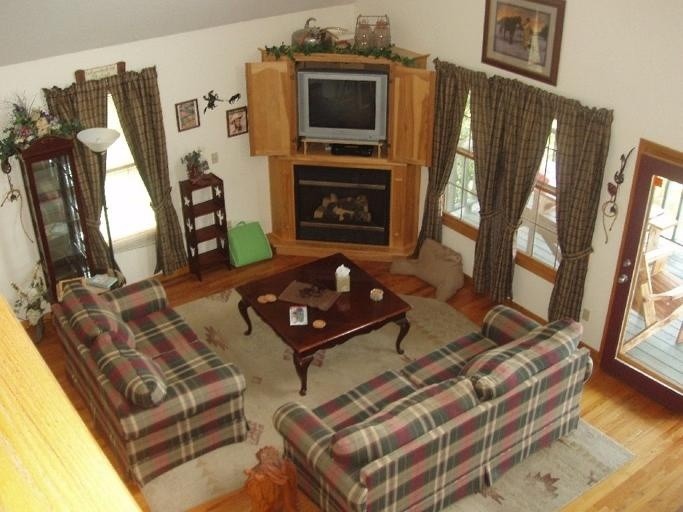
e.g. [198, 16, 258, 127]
[264, 156, 428, 262]
[17, 134, 97, 303]
[179, 171, 231, 282]
[246, 42, 438, 166]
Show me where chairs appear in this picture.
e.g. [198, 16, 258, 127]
[618, 256, 682, 356]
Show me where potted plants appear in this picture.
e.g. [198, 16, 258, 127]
[181, 150, 209, 184]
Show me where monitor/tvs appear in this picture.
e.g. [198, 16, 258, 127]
[298, 69, 388, 141]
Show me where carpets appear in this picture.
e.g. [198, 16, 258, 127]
[141, 288, 482, 512]
[439, 417, 637, 512]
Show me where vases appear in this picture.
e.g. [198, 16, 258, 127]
[32, 320, 44, 343]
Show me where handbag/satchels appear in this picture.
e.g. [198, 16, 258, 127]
[227, 220, 273, 267]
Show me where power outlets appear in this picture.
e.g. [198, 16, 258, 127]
[582, 308, 592, 322]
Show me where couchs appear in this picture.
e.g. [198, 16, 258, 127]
[271, 303, 593, 512]
[51, 278, 250, 486]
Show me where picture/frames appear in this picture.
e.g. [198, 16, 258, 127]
[226, 106, 248, 137]
[482, 0, 567, 86]
[175, 99, 200, 132]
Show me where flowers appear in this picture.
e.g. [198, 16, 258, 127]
[11, 258, 53, 327]
[0, 92, 80, 244]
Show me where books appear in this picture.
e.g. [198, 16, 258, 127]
[86, 274, 118, 289]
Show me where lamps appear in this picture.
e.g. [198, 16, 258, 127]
[76, 127, 121, 270]
